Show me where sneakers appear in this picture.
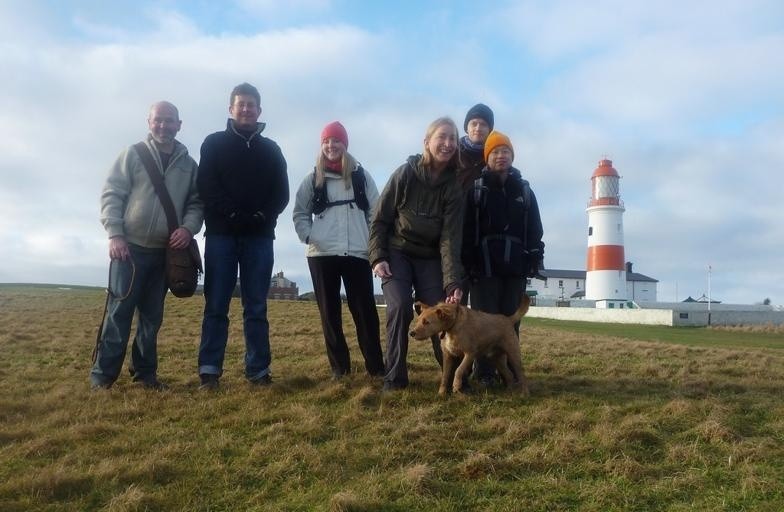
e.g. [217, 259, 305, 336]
[91, 382, 112, 392]
[330, 373, 409, 394]
[444, 370, 507, 394]
[199, 374, 219, 392]
[143, 376, 169, 392]
[255, 373, 274, 386]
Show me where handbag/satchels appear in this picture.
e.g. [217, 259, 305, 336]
[166, 239, 202, 298]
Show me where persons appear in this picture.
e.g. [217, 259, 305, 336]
[293, 121, 384, 377]
[368, 118, 473, 393]
[464, 131, 545, 386]
[459, 103, 494, 311]
[89, 101, 204, 391]
[197, 83, 290, 391]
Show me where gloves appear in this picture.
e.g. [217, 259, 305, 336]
[223, 211, 270, 238]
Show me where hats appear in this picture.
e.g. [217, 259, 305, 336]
[485, 130, 514, 163]
[465, 104, 494, 132]
[321, 122, 348, 149]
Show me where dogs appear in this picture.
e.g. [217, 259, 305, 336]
[409, 293, 531, 401]
